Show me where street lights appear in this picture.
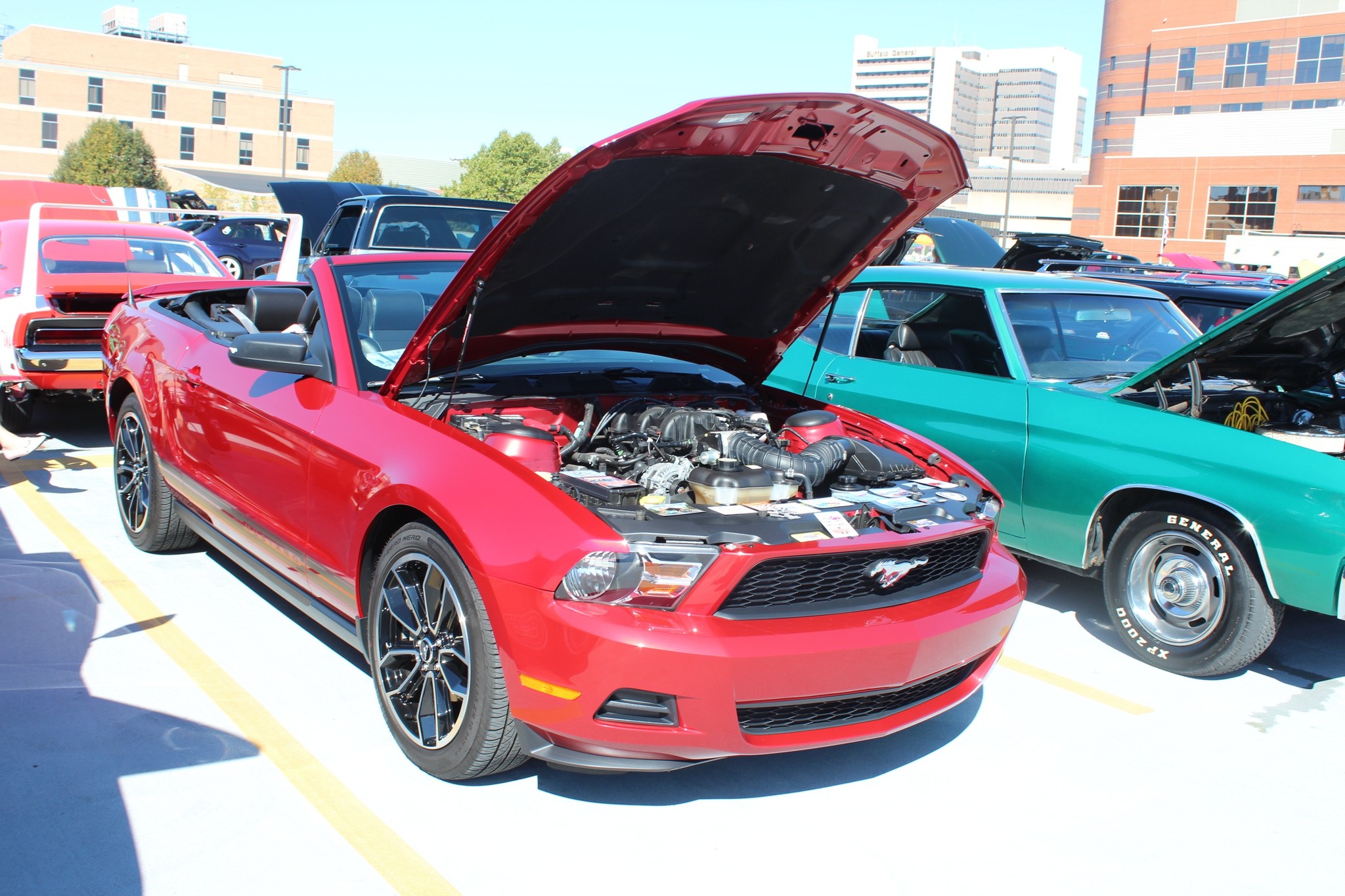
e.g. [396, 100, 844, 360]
[273, 65, 301, 174]
[1002, 116, 1027, 252]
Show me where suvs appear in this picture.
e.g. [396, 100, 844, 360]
[253, 181, 516, 276]
[1033, 259, 1286, 358]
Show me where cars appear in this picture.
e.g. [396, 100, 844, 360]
[101, 93, 1025, 778]
[762, 256, 1345, 676]
[127, 219, 208, 257]
[188, 216, 310, 280]
[0, 203, 304, 427]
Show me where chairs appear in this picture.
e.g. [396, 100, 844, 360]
[1012, 324, 1062, 364]
[241, 287, 308, 338]
[362, 287, 425, 352]
[882, 321, 968, 372]
